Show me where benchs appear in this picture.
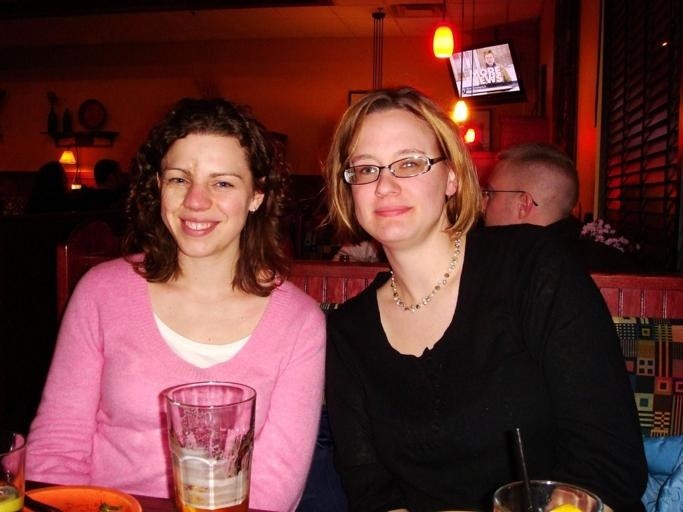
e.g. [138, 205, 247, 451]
[56, 232, 682, 512]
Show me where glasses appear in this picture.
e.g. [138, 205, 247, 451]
[344, 156, 444, 185]
[484, 190, 538, 207]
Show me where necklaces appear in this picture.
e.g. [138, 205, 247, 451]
[390, 231, 461, 312]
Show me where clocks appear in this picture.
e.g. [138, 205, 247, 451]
[78, 99, 106, 129]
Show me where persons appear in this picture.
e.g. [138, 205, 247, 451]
[24, 96, 328, 511]
[482, 141, 601, 272]
[481, 51, 512, 94]
[295, 81, 647, 512]
[27, 160, 121, 211]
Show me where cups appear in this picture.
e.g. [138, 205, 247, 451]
[164, 381, 257, 512]
[493, 480, 604, 512]
[0, 432, 26, 512]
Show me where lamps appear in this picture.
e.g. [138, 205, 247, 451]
[432, 0, 454, 59]
[58, 143, 80, 189]
[450, 0, 470, 124]
[464, 0, 475, 143]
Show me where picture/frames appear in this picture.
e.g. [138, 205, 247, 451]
[467, 107, 492, 152]
[348, 90, 368, 106]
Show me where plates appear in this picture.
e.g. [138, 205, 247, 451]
[24, 486, 142, 512]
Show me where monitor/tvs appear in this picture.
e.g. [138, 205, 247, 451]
[447, 39, 529, 104]
